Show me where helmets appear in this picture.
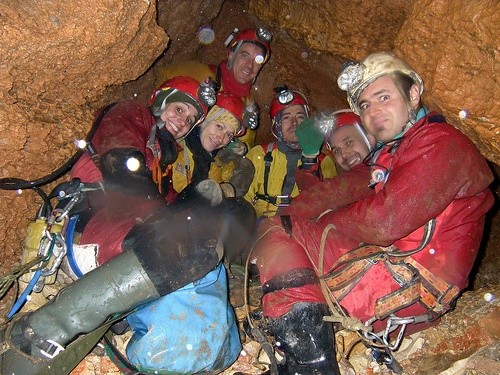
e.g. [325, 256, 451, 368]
[324, 112, 362, 146]
[347, 51, 424, 116]
[270, 93, 309, 119]
[231, 28, 271, 64]
[150, 77, 207, 124]
[215, 91, 246, 137]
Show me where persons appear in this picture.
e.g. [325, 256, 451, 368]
[293, 107, 375, 196]
[241, 84, 338, 220]
[158, 26, 274, 155]
[233, 50, 493, 375]
[170, 91, 264, 306]
[0, 76, 255, 375]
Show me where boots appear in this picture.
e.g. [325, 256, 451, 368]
[226, 264, 265, 308]
[0, 248, 161, 375]
[272, 302, 340, 375]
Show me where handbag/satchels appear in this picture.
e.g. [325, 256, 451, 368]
[127, 261, 242, 375]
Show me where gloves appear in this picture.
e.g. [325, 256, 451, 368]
[122, 152, 152, 192]
[297, 117, 324, 155]
[196, 178, 223, 205]
